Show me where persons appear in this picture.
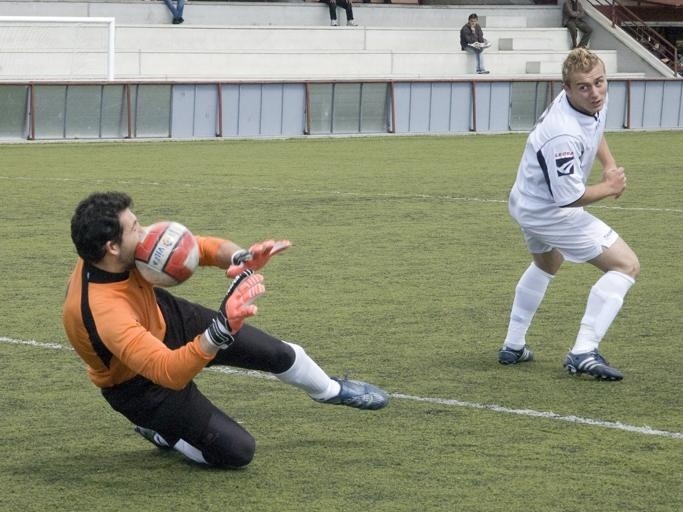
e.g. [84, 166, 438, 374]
[319, 0, 359, 27]
[561, 0, 593, 50]
[498, 45, 640, 379]
[460, 14, 490, 74]
[164, 0, 185, 24]
[61, 190, 390, 471]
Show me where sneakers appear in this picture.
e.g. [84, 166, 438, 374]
[563, 347, 624, 382]
[307, 376, 390, 411]
[347, 20, 358, 26]
[476, 70, 490, 75]
[498, 344, 536, 365]
[172, 16, 184, 24]
[135, 426, 167, 449]
[331, 20, 338, 26]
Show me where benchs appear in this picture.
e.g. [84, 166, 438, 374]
[0, 1, 647, 79]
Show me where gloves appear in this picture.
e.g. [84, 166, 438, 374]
[224, 239, 291, 280]
[205, 267, 265, 350]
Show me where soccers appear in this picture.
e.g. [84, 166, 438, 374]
[134, 221, 199, 287]
